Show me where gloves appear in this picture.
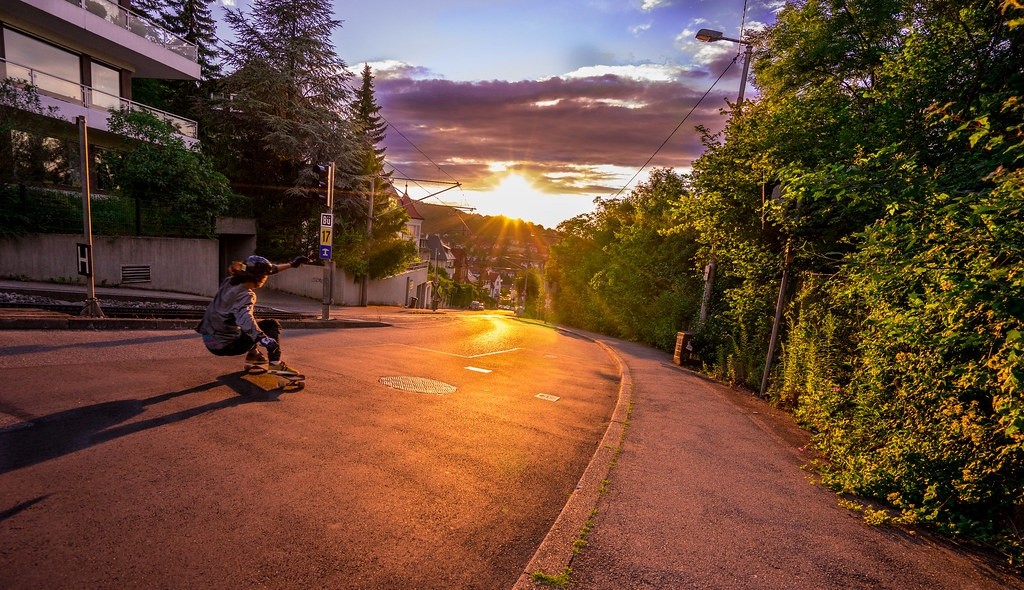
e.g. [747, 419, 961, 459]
[260, 336, 278, 352]
[291, 256, 310, 267]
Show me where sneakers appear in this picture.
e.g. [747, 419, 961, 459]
[245, 353, 270, 363]
[267, 361, 299, 375]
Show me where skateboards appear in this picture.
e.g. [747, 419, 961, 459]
[244, 362, 305, 389]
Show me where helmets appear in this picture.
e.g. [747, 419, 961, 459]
[243, 255, 276, 275]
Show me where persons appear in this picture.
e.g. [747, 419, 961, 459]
[200, 255, 310, 376]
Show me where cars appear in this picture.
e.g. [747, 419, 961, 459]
[498, 305, 524, 314]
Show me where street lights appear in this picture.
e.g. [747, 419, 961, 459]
[672, 29, 753, 366]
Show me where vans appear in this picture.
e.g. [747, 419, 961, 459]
[471, 301, 484, 311]
